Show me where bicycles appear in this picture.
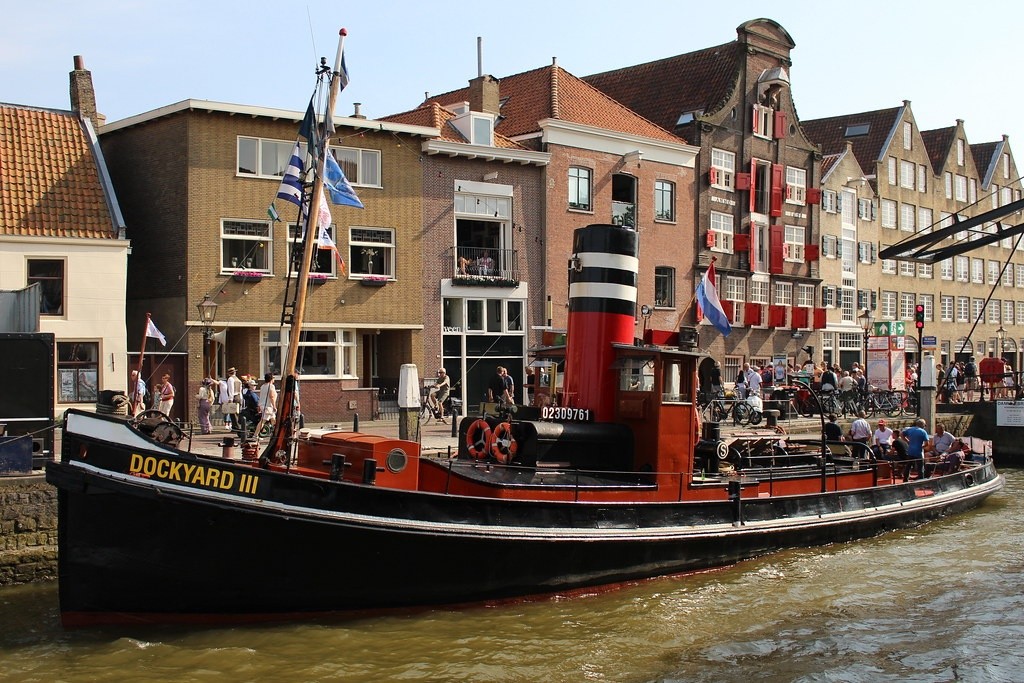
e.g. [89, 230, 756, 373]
[801, 386, 917, 419]
[698, 385, 755, 426]
[420, 385, 454, 425]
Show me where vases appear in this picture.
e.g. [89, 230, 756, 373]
[361, 281, 387, 286]
[233, 275, 262, 282]
[307, 279, 326, 284]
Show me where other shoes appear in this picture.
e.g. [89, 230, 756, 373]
[438, 418, 444, 422]
[224, 425, 231, 430]
[252, 436, 263, 441]
[433, 405, 439, 411]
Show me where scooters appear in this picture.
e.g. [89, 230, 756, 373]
[735, 386, 764, 425]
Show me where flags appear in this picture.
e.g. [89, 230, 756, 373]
[695, 264, 732, 337]
[146, 319, 166, 346]
[270, 101, 364, 277]
[339, 52, 350, 92]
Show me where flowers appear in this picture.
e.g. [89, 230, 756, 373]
[307, 274, 327, 279]
[362, 276, 387, 282]
[233, 271, 262, 277]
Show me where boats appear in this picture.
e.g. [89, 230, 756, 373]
[43, 28, 1003, 646]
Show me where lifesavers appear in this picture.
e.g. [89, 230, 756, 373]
[465, 419, 492, 460]
[490, 421, 518, 466]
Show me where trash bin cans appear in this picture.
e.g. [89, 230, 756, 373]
[774, 387, 798, 419]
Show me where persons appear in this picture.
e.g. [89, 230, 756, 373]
[851, 410, 872, 458]
[493, 366, 514, 404]
[711, 360, 867, 416]
[477, 251, 495, 276]
[873, 418, 965, 483]
[431, 368, 450, 422]
[194, 367, 301, 435]
[131, 370, 146, 420]
[150, 374, 175, 421]
[905, 355, 1014, 407]
[524, 366, 549, 406]
[822, 413, 844, 441]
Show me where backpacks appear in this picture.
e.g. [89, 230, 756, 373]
[763, 369, 773, 383]
[162, 385, 176, 398]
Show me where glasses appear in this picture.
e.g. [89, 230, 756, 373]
[438, 372, 444, 373]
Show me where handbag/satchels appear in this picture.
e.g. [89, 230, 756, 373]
[196, 400, 200, 408]
[222, 402, 240, 414]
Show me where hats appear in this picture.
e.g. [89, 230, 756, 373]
[1006, 365, 1011, 370]
[248, 380, 258, 386]
[754, 366, 759, 371]
[226, 367, 238, 374]
[768, 362, 773, 367]
[294, 369, 300, 373]
[878, 420, 887, 425]
[202, 378, 214, 387]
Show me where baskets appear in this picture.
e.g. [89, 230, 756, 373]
[420, 387, 429, 396]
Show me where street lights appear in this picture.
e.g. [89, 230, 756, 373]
[857, 309, 875, 393]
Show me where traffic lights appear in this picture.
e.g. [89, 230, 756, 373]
[915, 304, 924, 328]
